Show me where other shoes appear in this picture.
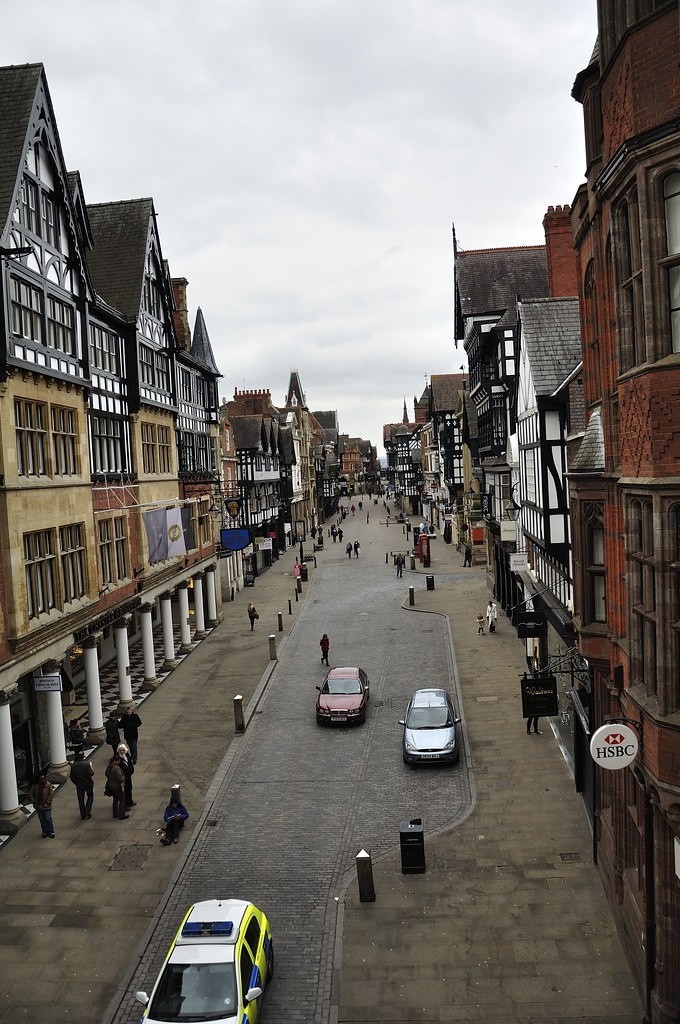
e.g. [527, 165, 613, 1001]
[397, 574, 402, 577]
[42, 834, 55, 838]
[326, 664, 330, 666]
[113, 815, 129, 820]
[81, 814, 92, 820]
[534, 731, 543, 735]
[160, 838, 178, 845]
[321, 658, 324, 664]
[123, 802, 136, 811]
[463, 566, 471, 567]
[478, 632, 480, 635]
[527, 730, 533, 734]
[482, 632, 485, 633]
[490, 631, 495, 635]
[130, 755, 137, 765]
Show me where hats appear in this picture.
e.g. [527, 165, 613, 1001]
[324, 634, 328, 638]
[109, 712, 116, 717]
[477, 613, 483, 618]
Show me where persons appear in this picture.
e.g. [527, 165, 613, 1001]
[248, 603, 256, 631]
[117, 744, 136, 811]
[70, 752, 94, 820]
[106, 711, 121, 756]
[320, 634, 330, 666]
[160, 797, 189, 846]
[475, 613, 485, 636]
[28, 772, 55, 838]
[120, 707, 142, 765]
[527, 717, 543, 734]
[395, 553, 404, 577]
[462, 546, 471, 567]
[486, 601, 497, 635]
[105, 755, 129, 820]
[69, 719, 84, 760]
[310, 489, 435, 558]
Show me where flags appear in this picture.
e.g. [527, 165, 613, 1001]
[181, 508, 196, 551]
[166, 507, 187, 557]
[142, 507, 169, 562]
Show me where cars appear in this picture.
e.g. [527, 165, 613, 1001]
[136, 898, 274, 1024]
[398, 688, 461, 765]
[314, 667, 369, 728]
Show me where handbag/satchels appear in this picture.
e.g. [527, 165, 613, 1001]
[354, 551, 356, 555]
[254, 613, 259, 619]
[311, 532, 313, 537]
[104, 782, 113, 796]
[342, 535, 343, 537]
[106, 735, 112, 745]
[357, 548, 361, 553]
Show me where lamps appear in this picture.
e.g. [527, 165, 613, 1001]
[467, 476, 480, 500]
[504, 481, 521, 521]
[199, 493, 219, 518]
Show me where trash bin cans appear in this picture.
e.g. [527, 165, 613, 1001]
[406, 523, 411, 531]
[426, 576, 434, 590]
[423, 554, 430, 567]
[301, 568, 308, 581]
[318, 537, 323, 545]
[399, 819, 425, 873]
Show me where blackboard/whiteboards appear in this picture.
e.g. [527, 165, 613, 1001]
[246, 571, 254, 583]
